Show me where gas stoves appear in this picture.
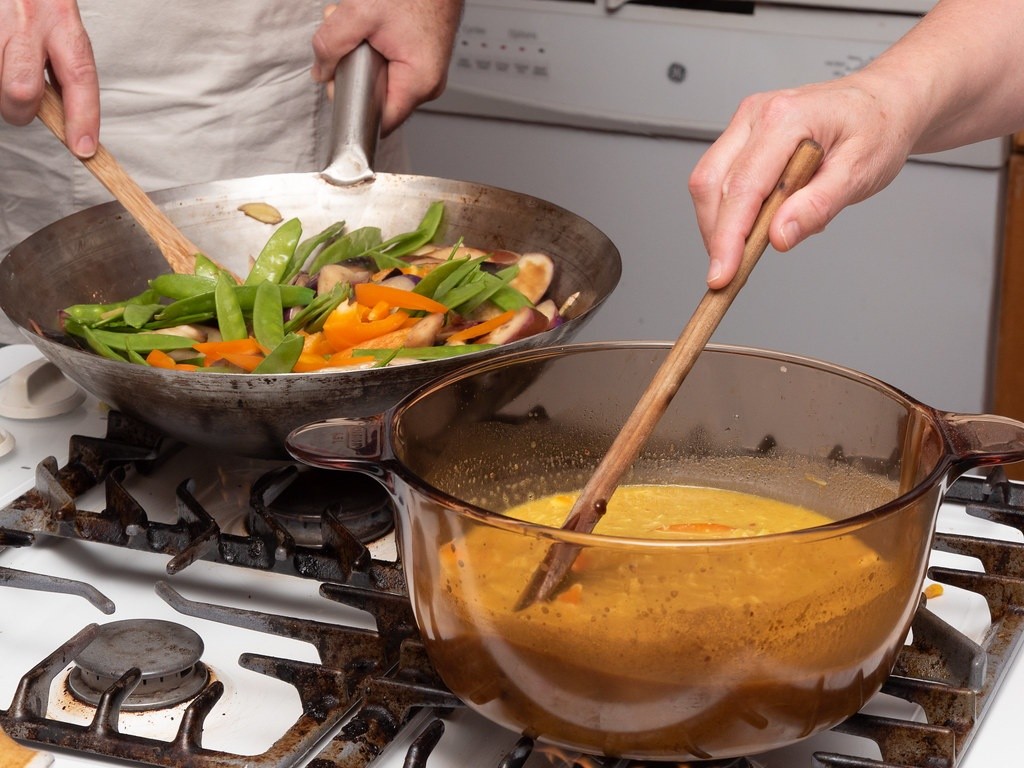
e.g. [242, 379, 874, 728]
[1, 405, 1021, 765]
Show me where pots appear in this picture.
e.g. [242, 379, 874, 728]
[0, 35, 623, 463]
[286, 342, 1022, 766]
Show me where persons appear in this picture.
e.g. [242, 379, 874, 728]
[688, 0, 1024, 290]
[0, 0, 463, 348]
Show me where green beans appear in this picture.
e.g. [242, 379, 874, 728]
[57, 197, 537, 375]
